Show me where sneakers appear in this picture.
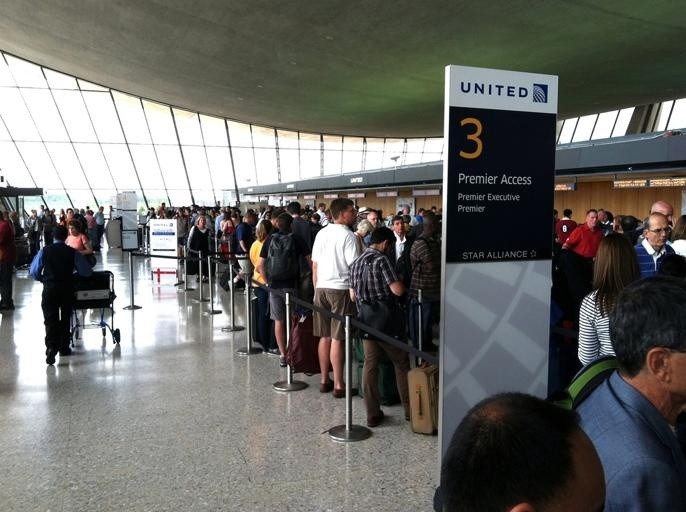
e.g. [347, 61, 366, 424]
[46, 344, 72, 364]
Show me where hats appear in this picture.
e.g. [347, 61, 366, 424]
[359, 207, 372, 214]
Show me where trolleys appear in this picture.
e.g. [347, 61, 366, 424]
[13, 234, 29, 272]
[69, 270, 121, 346]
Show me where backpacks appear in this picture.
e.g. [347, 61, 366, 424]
[265, 232, 300, 289]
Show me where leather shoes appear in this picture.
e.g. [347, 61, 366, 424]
[332, 388, 358, 398]
[367, 410, 384, 426]
[320, 379, 334, 392]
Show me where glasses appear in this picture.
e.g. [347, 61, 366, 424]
[644, 227, 672, 233]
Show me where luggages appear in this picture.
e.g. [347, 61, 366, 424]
[354, 330, 402, 406]
[408, 287, 440, 434]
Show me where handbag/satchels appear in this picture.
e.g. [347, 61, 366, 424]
[284, 310, 333, 375]
[355, 298, 407, 342]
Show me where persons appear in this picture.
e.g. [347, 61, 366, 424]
[0, 204, 105, 366]
[147, 198, 441, 428]
[440, 390, 606, 512]
[548, 198, 685, 389]
[568, 273, 686, 511]
